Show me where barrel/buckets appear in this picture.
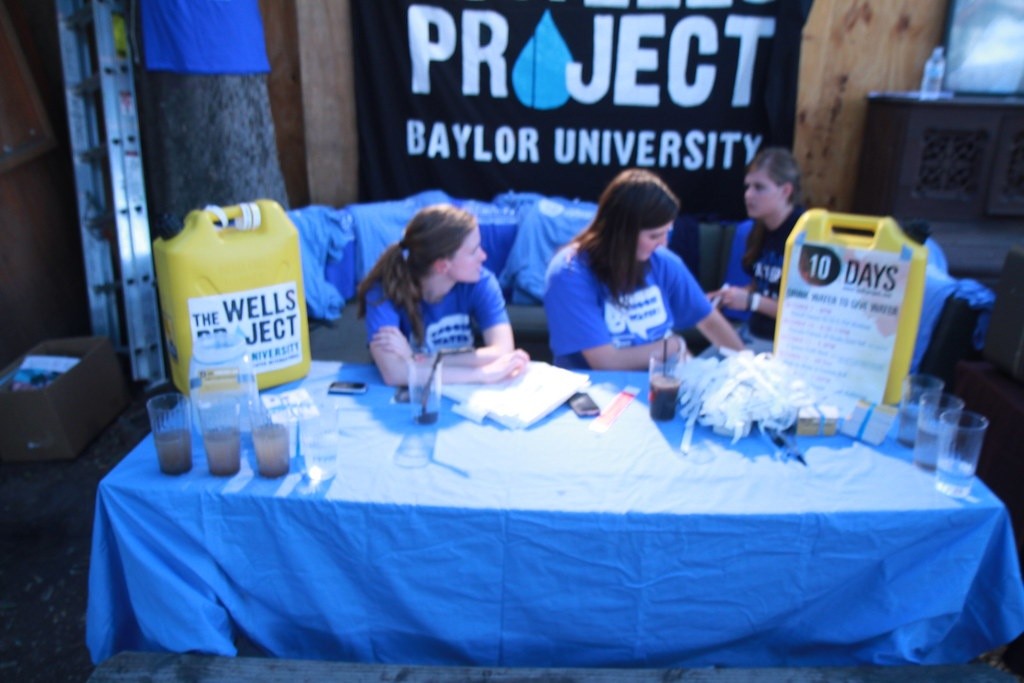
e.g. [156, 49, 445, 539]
[768, 206, 931, 406]
[153, 198, 312, 397]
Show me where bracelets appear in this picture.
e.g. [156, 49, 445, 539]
[747, 291, 761, 312]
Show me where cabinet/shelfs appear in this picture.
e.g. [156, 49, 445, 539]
[864, 92, 1023, 221]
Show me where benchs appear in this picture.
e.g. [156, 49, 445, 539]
[290, 191, 979, 380]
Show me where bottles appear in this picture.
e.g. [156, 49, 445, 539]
[186, 333, 261, 436]
[919, 45, 946, 101]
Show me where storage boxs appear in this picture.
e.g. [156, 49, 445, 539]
[0, 336, 133, 461]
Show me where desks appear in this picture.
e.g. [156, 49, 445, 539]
[84, 360, 1023, 664]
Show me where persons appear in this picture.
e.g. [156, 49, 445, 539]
[701, 150, 804, 362]
[357, 204, 528, 386]
[545, 170, 744, 372]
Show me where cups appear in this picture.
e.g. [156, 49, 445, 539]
[242, 394, 295, 478]
[146, 393, 193, 475]
[194, 390, 242, 477]
[897, 372, 946, 448]
[934, 409, 990, 500]
[649, 347, 683, 421]
[406, 355, 443, 426]
[912, 392, 964, 473]
